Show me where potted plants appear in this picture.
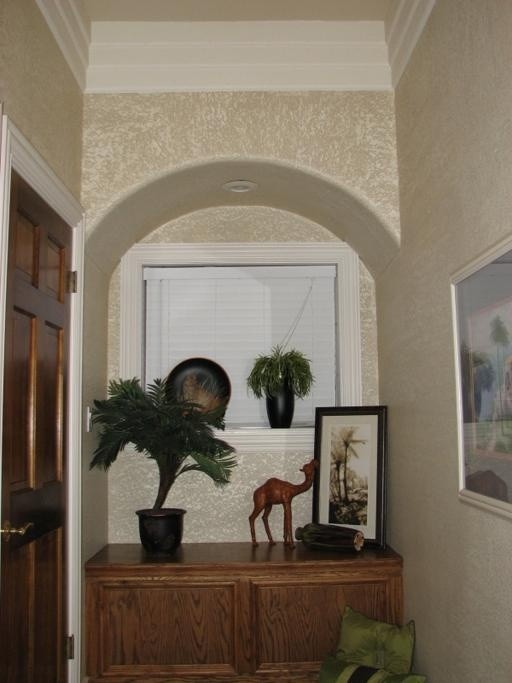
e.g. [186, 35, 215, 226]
[87, 375, 239, 558]
[244, 344, 315, 430]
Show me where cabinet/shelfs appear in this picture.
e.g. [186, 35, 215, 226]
[79, 540, 408, 681]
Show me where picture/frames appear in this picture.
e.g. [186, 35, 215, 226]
[309, 402, 391, 551]
[446, 228, 512, 525]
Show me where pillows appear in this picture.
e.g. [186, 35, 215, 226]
[315, 601, 435, 681]
